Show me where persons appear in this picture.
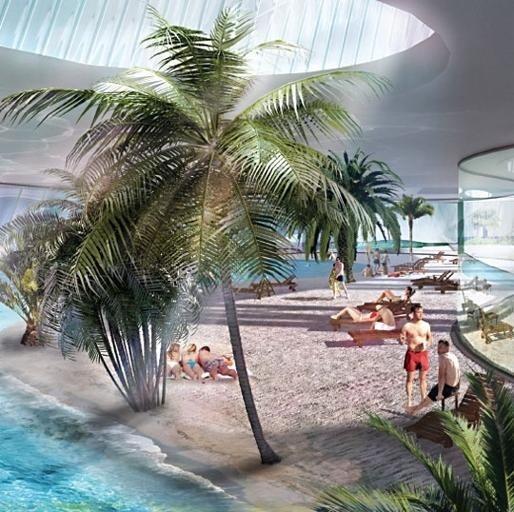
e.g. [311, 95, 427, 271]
[396, 304, 432, 407]
[328, 246, 493, 331]
[401, 340, 459, 417]
[197, 346, 238, 382]
[179, 344, 204, 383]
[157, 342, 182, 381]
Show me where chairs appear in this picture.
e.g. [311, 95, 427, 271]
[233, 251, 514, 347]
[403, 373, 506, 448]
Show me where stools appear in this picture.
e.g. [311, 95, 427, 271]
[440, 392, 460, 411]
